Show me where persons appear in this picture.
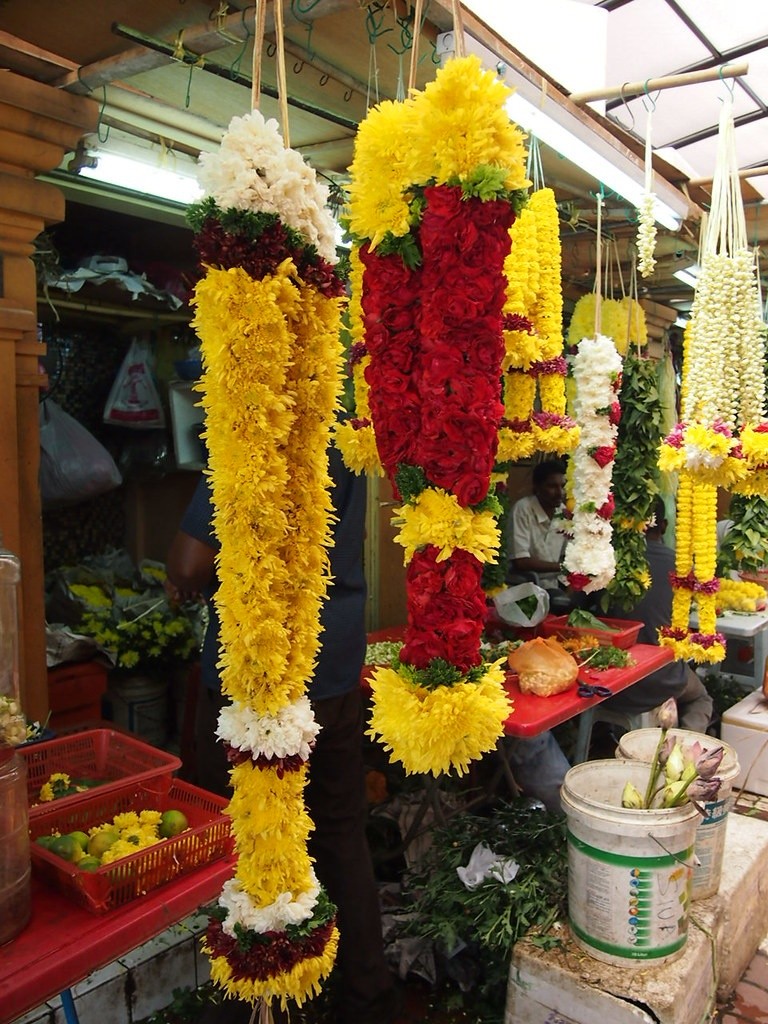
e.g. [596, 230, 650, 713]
[716, 498, 738, 552]
[164, 414, 380, 1023]
[443, 732, 571, 823]
[507, 458, 768, 734]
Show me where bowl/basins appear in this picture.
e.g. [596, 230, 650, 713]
[175, 359, 205, 379]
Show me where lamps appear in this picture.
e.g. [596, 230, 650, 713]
[69, 135, 205, 207]
[436, 31, 691, 233]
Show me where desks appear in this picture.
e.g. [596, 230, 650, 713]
[689, 600, 768, 692]
[358, 624, 675, 769]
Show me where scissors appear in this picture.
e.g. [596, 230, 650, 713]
[574, 678, 612, 697]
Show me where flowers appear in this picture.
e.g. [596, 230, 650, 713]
[47, 559, 195, 670]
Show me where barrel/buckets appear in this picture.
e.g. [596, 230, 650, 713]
[193, 422, 209, 463]
[559, 759, 705, 968]
[0, 744, 33, 947]
[615, 728, 739, 901]
[103, 676, 170, 749]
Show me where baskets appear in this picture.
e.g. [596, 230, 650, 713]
[540, 617, 645, 650]
[28, 773, 237, 915]
[13, 727, 182, 816]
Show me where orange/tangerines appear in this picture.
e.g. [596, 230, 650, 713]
[76, 857, 101, 873]
[49, 836, 81, 862]
[159, 810, 188, 836]
[36, 836, 52, 846]
[69, 831, 90, 850]
[87, 832, 119, 858]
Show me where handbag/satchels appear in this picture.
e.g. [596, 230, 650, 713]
[35, 391, 125, 508]
[508, 633, 579, 698]
[101, 337, 169, 431]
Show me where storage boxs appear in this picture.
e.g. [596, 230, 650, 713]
[504, 812, 768, 1024]
[29, 780, 239, 916]
[544, 614, 645, 650]
[721, 686, 768, 797]
[16, 729, 182, 817]
